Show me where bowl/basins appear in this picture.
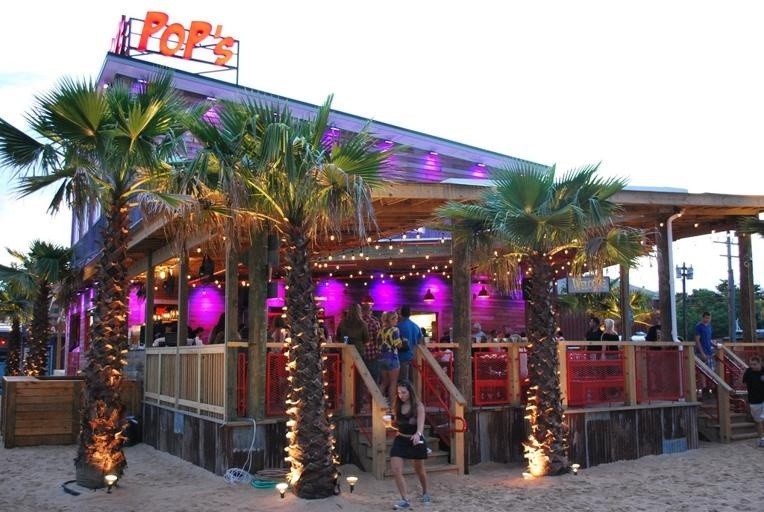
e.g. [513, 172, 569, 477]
[492, 338, 512, 343]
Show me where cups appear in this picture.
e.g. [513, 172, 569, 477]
[327, 335, 332, 342]
[343, 336, 348, 343]
[187, 339, 194, 346]
[476, 336, 481, 343]
[383, 415, 392, 428]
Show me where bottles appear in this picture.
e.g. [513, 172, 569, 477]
[195, 337, 202, 346]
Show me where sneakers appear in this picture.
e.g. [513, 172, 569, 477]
[394, 498, 410, 509]
[423, 492, 430, 503]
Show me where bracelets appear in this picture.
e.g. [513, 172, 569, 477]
[417, 431, 423, 437]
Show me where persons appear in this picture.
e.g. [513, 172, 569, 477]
[694, 310, 714, 399]
[579, 318, 621, 398]
[742, 354, 764, 450]
[384, 379, 431, 510]
[420, 322, 564, 378]
[151, 311, 331, 347]
[645, 314, 661, 392]
[335, 303, 424, 409]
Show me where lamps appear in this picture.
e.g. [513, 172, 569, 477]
[423, 288, 436, 302]
[478, 286, 490, 297]
[361, 287, 375, 305]
